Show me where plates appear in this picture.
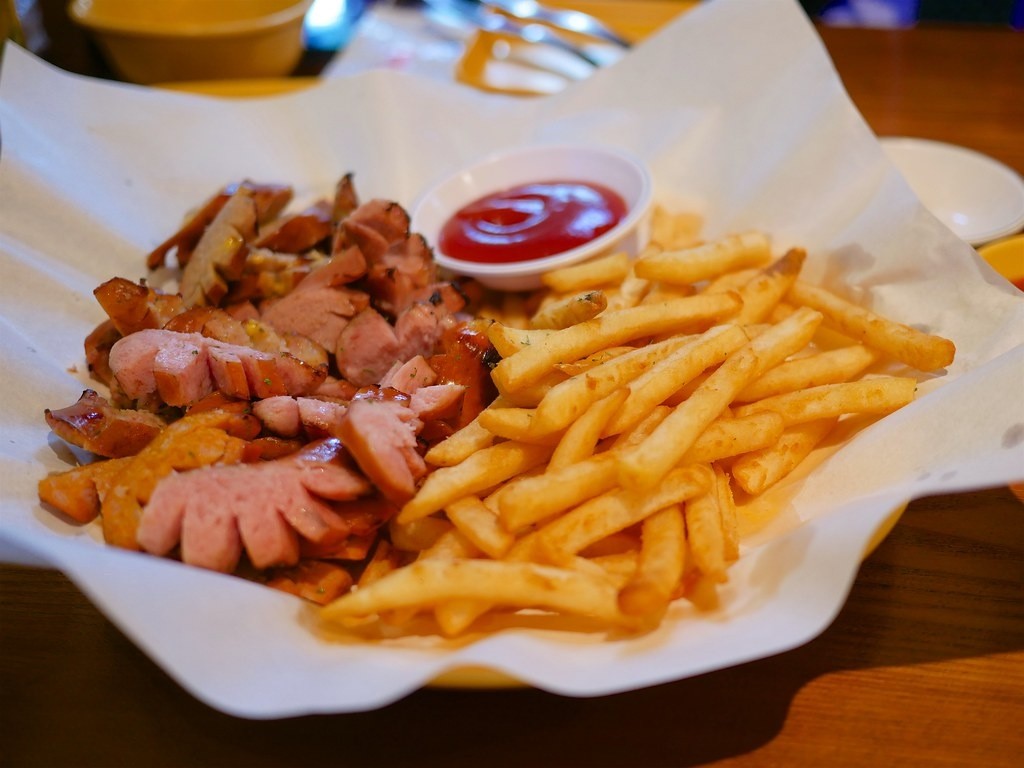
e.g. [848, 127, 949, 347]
[881, 137, 1024, 249]
[427, 497, 917, 692]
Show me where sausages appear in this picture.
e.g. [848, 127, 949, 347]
[38, 176, 489, 598]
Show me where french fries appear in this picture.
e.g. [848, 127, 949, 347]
[318, 213, 958, 633]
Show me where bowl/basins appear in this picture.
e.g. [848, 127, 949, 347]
[69, 0, 313, 79]
[411, 145, 648, 294]
